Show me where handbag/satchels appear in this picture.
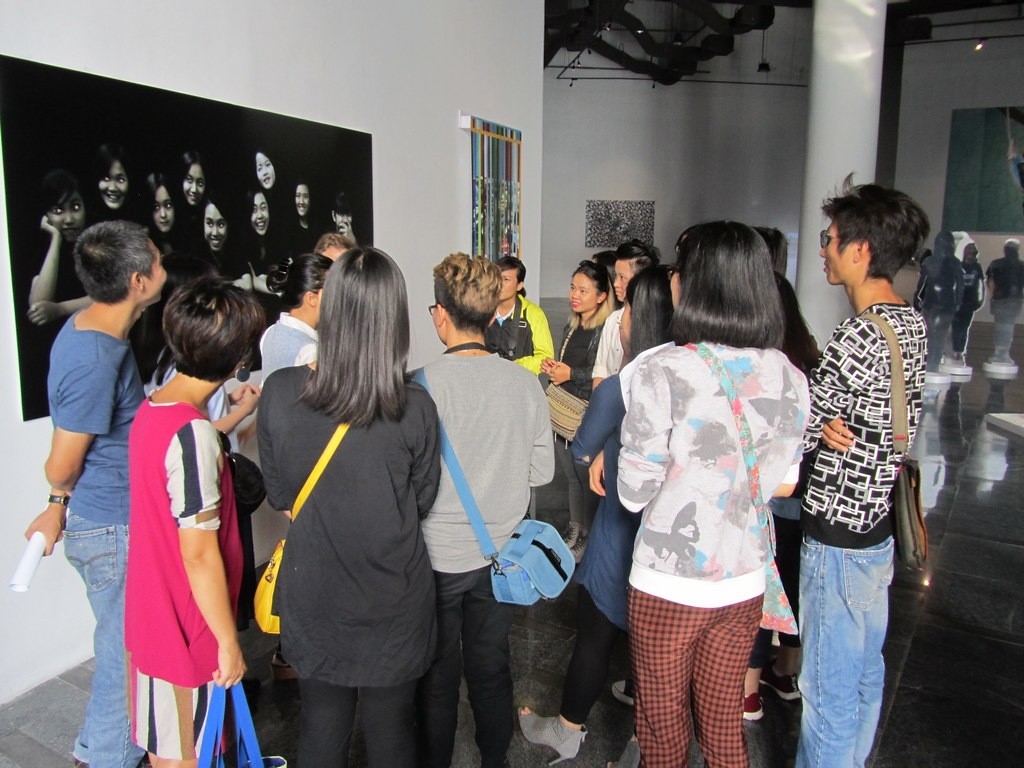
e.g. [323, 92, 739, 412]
[254, 539, 281, 634]
[227, 451, 267, 524]
[491, 519, 575, 605]
[545, 381, 590, 442]
[895, 458, 930, 573]
[757, 549, 799, 636]
[198, 677, 288, 768]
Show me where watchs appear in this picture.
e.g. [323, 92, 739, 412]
[47, 495, 70, 504]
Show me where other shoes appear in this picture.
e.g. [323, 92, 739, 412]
[612, 680, 635, 706]
[72, 754, 152, 768]
[742, 692, 764, 721]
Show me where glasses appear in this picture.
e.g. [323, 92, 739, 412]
[666, 264, 681, 279]
[428, 305, 449, 316]
[820, 230, 845, 248]
[219, 430, 238, 476]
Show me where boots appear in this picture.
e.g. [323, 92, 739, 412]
[570, 529, 590, 564]
[562, 520, 582, 550]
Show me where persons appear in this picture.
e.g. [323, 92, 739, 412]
[256, 247, 443, 767]
[792, 174, 931, 768]
[618, 224, 809, 768]
[24, 222, 167, 767]
[486, 226, 819, 721]
[402, 254, 556, 768]
[125, 278, 254, 767]
[516, 266, 685, 767]
[153, 233, 354, 680]
[27, 144, 363, 334]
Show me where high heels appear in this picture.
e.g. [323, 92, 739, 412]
[517, 707, 587, 765]
[606, 740, 641, 768]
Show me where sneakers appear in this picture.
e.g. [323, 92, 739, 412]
[759, 658, 802, 699]
